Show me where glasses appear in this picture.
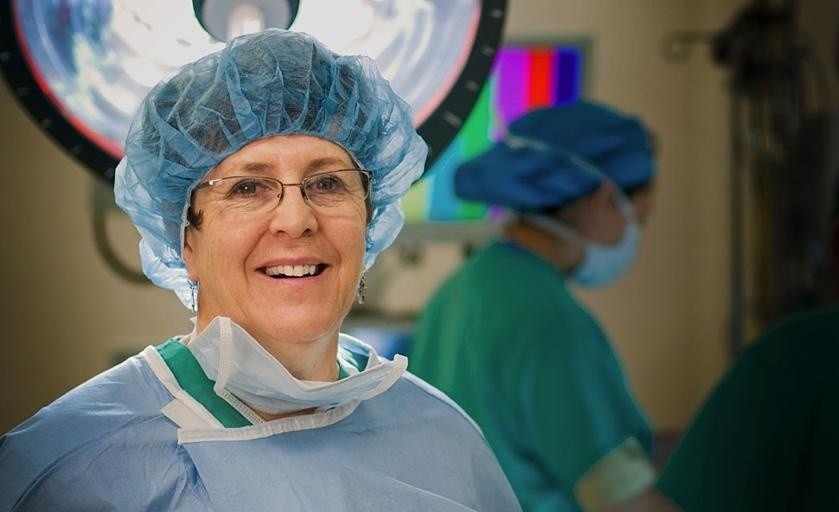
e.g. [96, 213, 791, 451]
[186, 167, 374, 214]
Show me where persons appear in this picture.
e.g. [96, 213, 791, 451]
[0, 29, 524, 509]
[402, 102, 681, 509]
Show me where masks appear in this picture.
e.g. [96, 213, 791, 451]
[570, 220, 642, 291]
[185, 315, 409, 416]
